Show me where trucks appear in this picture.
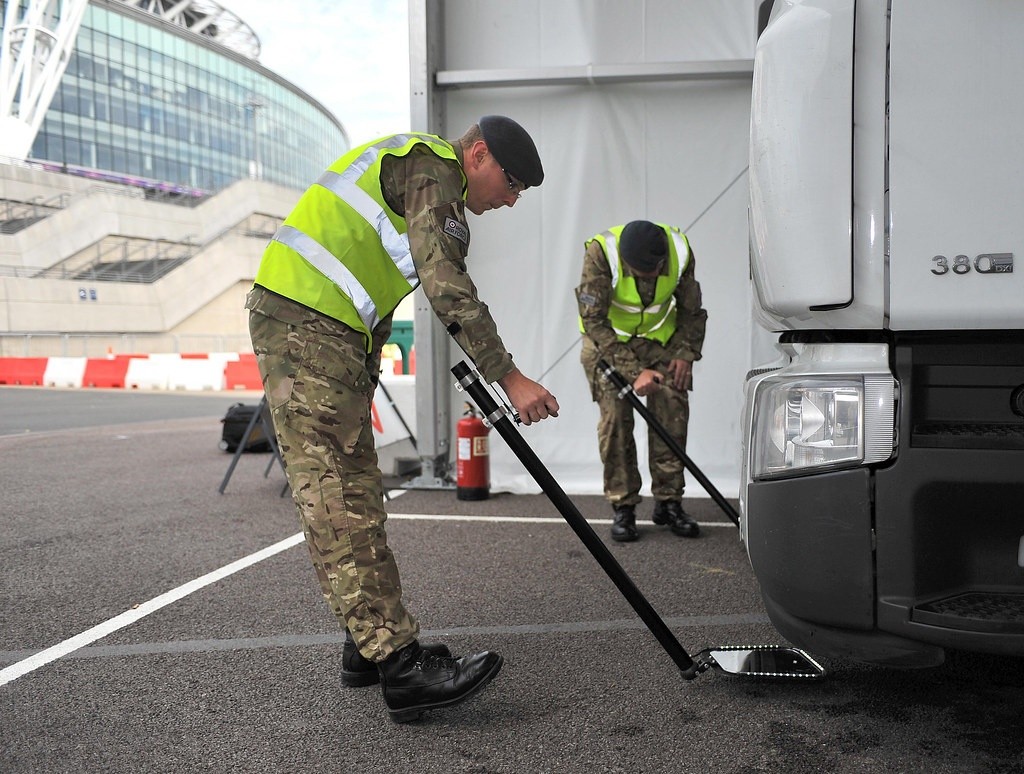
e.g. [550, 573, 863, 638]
[740, 0, 1024, 659]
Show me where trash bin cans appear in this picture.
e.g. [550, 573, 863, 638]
[386, 320, 416, 374]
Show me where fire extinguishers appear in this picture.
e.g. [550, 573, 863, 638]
[456, 400, 493, 499]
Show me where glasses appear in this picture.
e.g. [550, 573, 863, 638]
[499, 162, 522, 200]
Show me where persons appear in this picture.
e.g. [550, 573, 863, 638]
[244, 115, 548, 720]
[575, 221, 709, 541]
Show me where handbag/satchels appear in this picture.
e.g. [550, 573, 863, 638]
[221, 403, 278, 453]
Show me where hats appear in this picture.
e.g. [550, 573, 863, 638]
[619, 220, 669, 273]
[478, 115, 544, 186]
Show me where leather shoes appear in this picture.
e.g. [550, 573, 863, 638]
[340, 623, 451, 689]
[652, 498, 700, 539]
[377, 640, 504, 725]
[610, 503, 639, 543]
